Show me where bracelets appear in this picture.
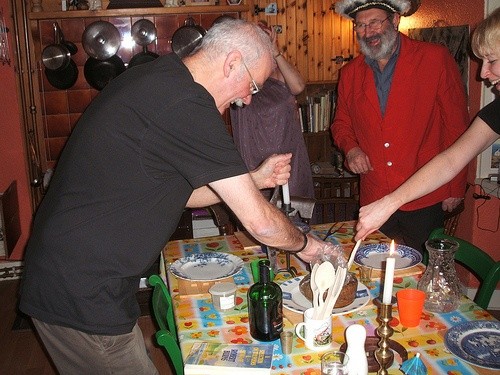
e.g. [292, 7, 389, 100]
[296, 233, 307, 253]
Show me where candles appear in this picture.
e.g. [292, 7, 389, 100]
[383, 237, 396, 306]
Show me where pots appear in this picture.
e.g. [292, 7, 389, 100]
[40, 21, 79, 90]
[82, 19, 126, 91]
[171, 16, 207, 59]
[128, 18, 160, 70]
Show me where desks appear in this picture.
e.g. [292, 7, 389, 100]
[308, 159, 361, 227]
[160, 221, 500, 375]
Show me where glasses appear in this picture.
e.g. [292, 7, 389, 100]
[242, 60, 260, 95]
[353, 12, 395, 33]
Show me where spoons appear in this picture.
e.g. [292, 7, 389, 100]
[310, 261, 336, 320]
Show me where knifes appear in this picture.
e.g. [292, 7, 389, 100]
[348, 238, 362, 271]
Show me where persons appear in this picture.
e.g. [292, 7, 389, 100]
[211, 16, 315, 223]
[17, 18, 337, 375]
[330, 0, 468, 255]
[355, 7, 500, 241]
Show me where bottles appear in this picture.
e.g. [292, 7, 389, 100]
[247, 260, 283, 343]
[417, 238, 464, 314]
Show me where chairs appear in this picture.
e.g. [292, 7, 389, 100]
[423, 228, 500, 313]
[148, 274, 184, 375]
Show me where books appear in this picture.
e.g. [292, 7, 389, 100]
[297, 90, 337, 133]
[184, 343, 274, 375]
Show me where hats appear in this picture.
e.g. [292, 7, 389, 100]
[334, 0, 421, 19]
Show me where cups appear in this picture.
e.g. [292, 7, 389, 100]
[208, 282, 237, 312]
[321, 351, 349, 375]
[280, 331, 293, 354]
[359, 266, 372, 283]
[295, 308, 333, 351]
[396, 288, 425, 327]
[250, 258, 274, 283]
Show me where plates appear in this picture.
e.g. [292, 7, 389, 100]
[340, 336, 408, 372]
[353, 242, 422, 271]
[170, 251, 244, 282]
[279, 276, 371, 316]
[444, 320, 500, 371]
[291, 221, 311, 234]
